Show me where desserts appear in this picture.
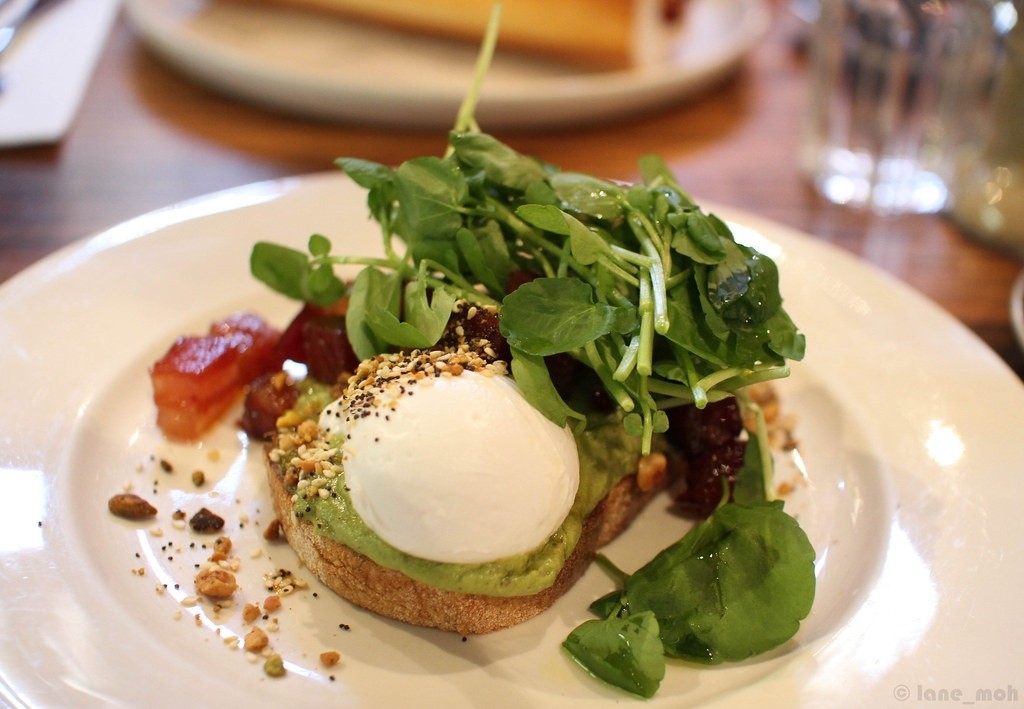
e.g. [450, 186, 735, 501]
[267, 336, 665, 638]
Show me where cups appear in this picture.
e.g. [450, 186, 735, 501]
[789, 2, 1005, 218]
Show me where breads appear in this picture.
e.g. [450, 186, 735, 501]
[294, 0, 638, 69]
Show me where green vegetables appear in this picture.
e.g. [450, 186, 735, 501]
[243, 1, 817, 703]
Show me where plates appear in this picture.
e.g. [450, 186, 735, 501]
[121, 0, 770, 127]
[2, 0, 120, 146]
[1, 171, 1023, 708]
